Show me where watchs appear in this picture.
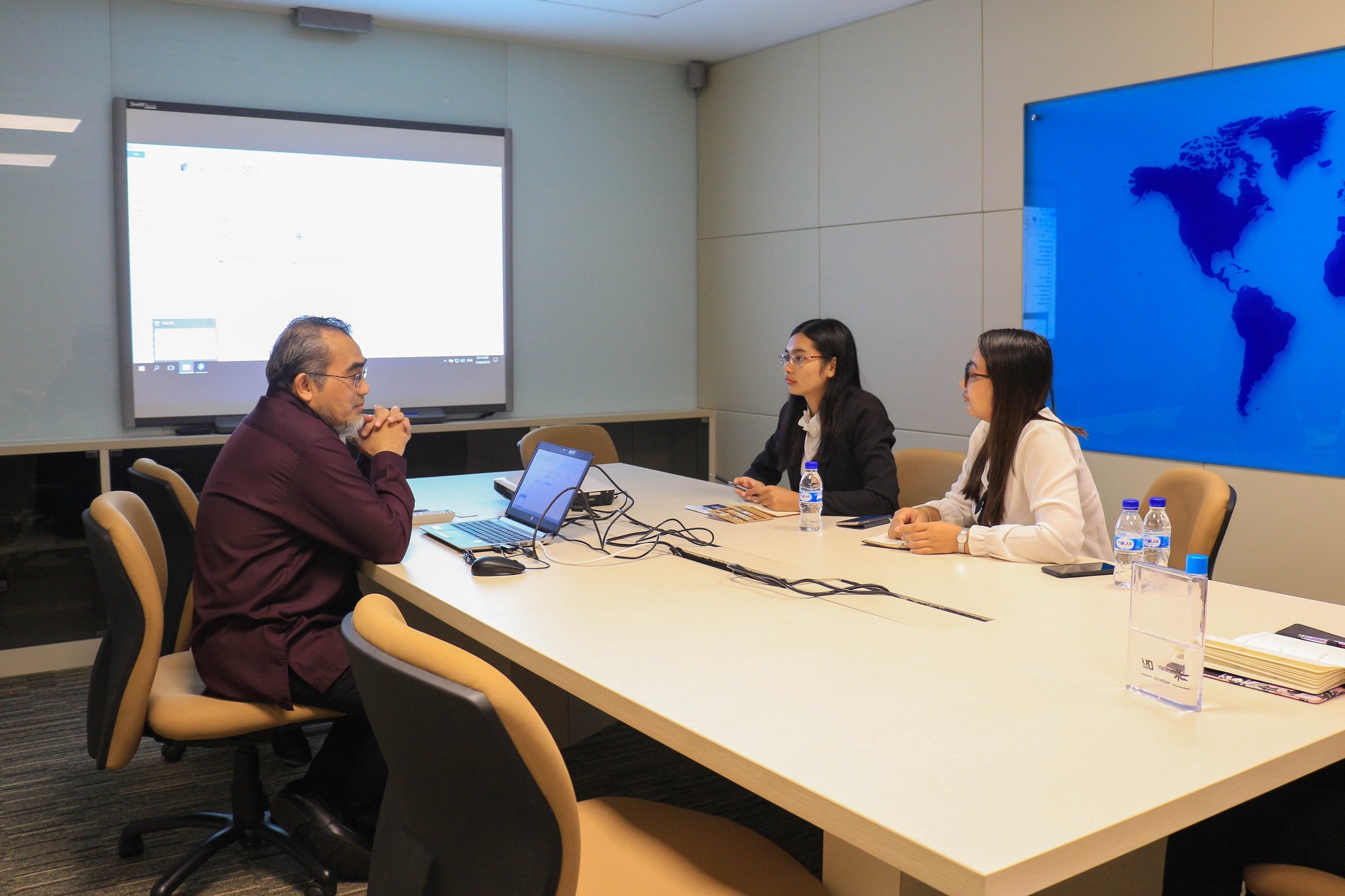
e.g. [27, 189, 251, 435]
[955, 527, 968, 554]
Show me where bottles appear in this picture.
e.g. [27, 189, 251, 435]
[1143, 497, 1172, 589]
[1126, 553, 1210, 712]
[1113, 499, 1145, 589]
[799, 461, 824, 532]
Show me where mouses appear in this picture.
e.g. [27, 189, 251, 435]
[470, 555, 525, 576]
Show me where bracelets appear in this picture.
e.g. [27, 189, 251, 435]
[920, 507, 931, 522]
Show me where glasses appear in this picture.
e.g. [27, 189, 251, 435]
[964, 362, 992, 389]
[778, 353, 822, 366]
[304, 367, 368, 388]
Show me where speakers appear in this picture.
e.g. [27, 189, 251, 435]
[689, 63, 706, 88]
[292, 6, 372, 34]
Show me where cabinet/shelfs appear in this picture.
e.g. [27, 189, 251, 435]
[0, 409, 716, 650]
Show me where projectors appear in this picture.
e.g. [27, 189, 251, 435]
[493, 472, 614, 507]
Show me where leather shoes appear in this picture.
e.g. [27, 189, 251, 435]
[279, 778, 379, 882]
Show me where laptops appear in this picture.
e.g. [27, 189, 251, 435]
[419, 441, 595, 552]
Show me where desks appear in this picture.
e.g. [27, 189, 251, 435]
[362, 459, 1345, 896]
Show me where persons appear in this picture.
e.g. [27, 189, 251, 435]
[187, 314, 412, 882]
[733, 319, 900, 515]
[888, 328, 1115, 564]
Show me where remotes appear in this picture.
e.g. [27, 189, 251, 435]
[412, 509, 455, 525]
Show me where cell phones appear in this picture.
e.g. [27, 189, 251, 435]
[836, 514, 893, 526]
[1041, 562, 1114, 578]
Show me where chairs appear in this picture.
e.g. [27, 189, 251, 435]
[340, 591, 834, 896]
[894, 450, 971, 510]
[518, 425, 620, 471]
[126, 458, 310, 764]
[1138, 468, 1237, 580]
[80, 491, 349, 896]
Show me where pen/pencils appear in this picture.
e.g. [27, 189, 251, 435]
[709, 473, 758, 497]
[1297, 634, 1345, 649]
[888, 518, 914, 542]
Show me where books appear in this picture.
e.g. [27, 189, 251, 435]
[1203, 623, 1345, 704]
[860, 532, 910, 552]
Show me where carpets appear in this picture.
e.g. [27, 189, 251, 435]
[0, 665, 368, 896]
